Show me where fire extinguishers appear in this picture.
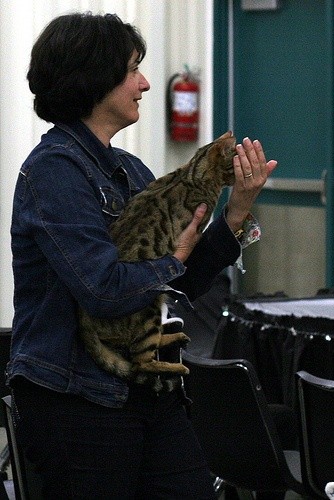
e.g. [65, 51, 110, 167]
[166, 64, 202, 144]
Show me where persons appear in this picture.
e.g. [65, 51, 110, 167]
[6, 12, 277, 500]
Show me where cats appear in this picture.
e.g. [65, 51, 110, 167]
[80, 130, 238, 391]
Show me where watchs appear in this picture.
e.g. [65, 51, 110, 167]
[232, 229, 247, 243]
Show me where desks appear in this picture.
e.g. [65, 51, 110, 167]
[221, 293, 333, 446]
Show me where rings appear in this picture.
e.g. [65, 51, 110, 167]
[244, 173, 253, 177]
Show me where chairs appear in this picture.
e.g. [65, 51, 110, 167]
[178, 352, 302, 499]
[291, 334, 334, 500]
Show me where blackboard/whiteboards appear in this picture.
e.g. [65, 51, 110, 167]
[228, 1, 334, 194]
